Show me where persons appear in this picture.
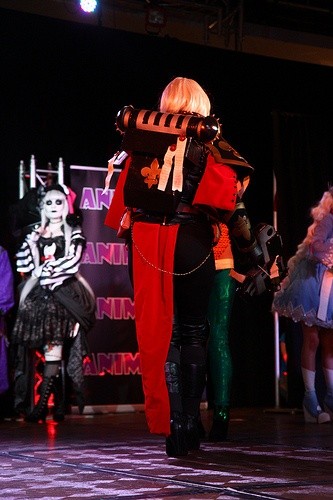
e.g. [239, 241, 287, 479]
[102, 76, 332, 458]
[16, 179, 99, 428]
[0, 245, 16, 397]
